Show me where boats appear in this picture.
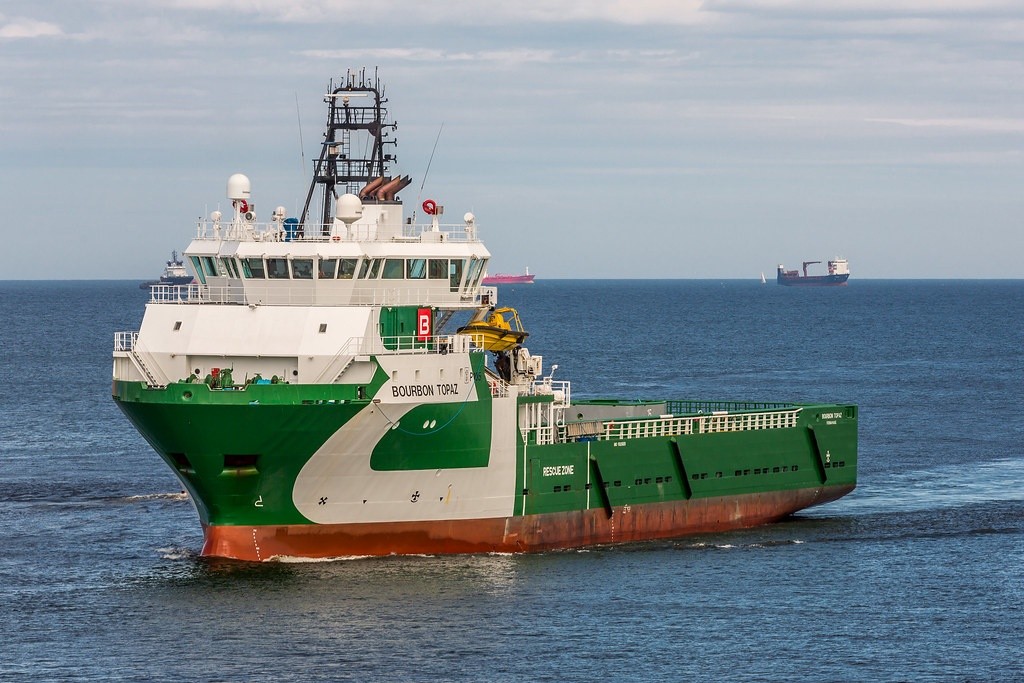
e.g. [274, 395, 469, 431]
[139, 249, 197, 290]
[481, 270, 535, 285]
[458, 308, 530, 352]
[110, 65, 858, 563]
[777, 260, 850, 285]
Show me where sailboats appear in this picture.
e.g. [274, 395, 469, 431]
[761, 273, 766, 284]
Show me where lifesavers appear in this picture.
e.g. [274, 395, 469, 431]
[233, 200, 248, 213]
[423, 200, 437, 214]
